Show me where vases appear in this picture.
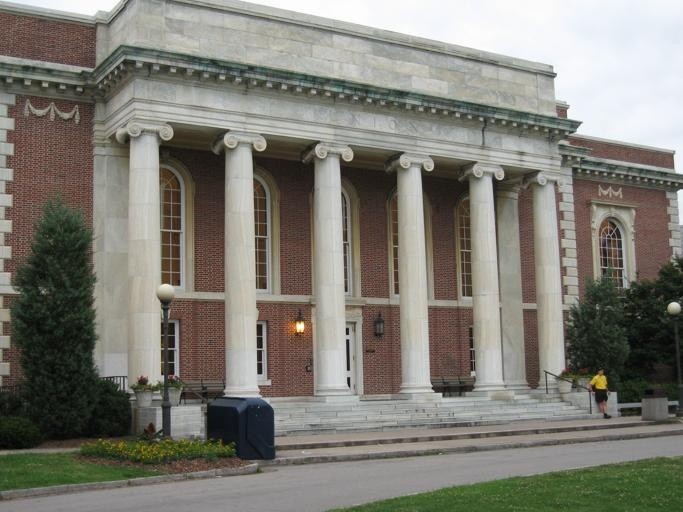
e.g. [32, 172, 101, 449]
[556, 376, 590, 393]
[134, 387, 183, 407]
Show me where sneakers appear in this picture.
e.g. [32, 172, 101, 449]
[604, 414, 611, 418]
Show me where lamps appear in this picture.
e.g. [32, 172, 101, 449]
[374, 311, 384, 338]
[294, 309, 305, 336]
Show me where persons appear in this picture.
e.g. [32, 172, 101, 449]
[588, 369, 612, 419]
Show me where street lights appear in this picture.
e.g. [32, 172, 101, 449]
[668, 302, 683, 417]
[156, 284, 175, 436]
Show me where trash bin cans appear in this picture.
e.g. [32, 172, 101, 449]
[641, 387, 668, 421]
[207, 396, 276, 460]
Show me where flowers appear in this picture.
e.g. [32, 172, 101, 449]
[130, 374, 185, 392]
[558, 368, 593, 379]
